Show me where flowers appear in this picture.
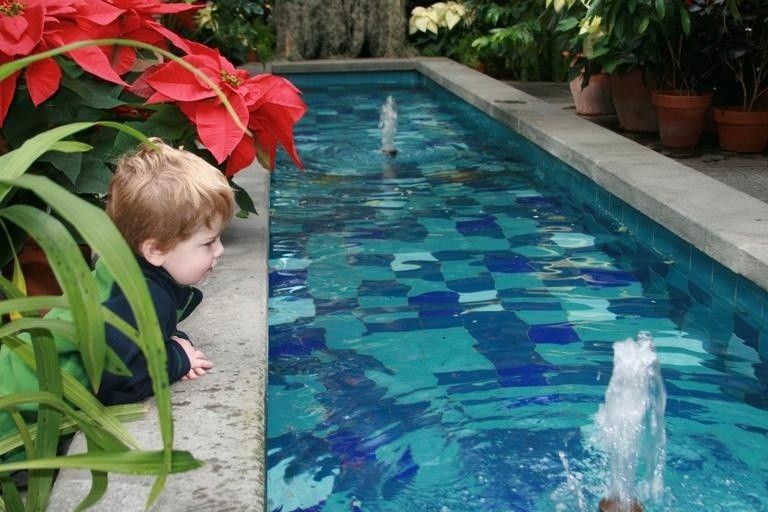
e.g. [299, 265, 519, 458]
[0, 0, 308, 262]
[408, 1, 479, 37]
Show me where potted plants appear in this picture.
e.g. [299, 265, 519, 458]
[545, 1, 768, 160]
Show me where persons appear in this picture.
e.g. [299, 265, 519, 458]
[0, 136, 237, 476]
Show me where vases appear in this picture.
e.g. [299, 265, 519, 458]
[6, 230, 92, 318]
